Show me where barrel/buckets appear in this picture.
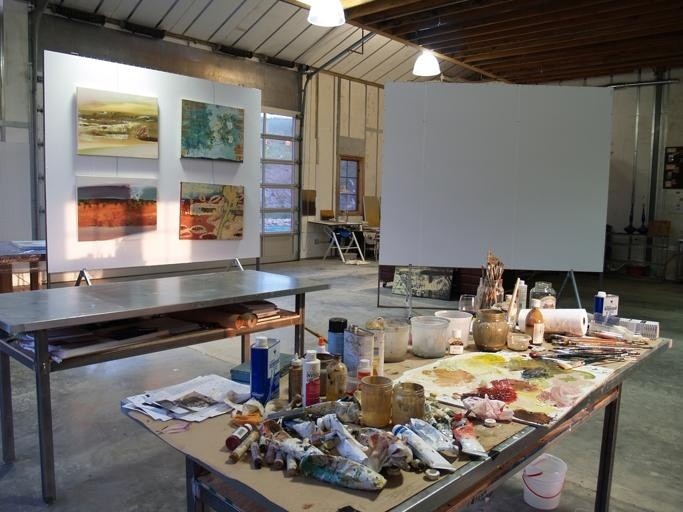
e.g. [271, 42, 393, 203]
[361, 376, 394, 428]
[522, 453, 569, 510]
[384, 321, 410, 363]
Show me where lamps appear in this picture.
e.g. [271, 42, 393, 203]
[305, 0, 347, 29]
[410, 50, 441, 77]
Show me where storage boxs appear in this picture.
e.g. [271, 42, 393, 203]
[648, 220, 671, 236]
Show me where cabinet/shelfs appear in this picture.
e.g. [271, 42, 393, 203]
[609, 230, 670, 283]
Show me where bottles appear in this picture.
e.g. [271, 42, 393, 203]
[471, 310, 508, 352]
[474, 276, 505, 308]
[450, 330, 464, 354]
[356, 359, 371, 384]
[502, 279, 556, 345]
[327, 317, 347, 365]
[288, 335, 349, 405]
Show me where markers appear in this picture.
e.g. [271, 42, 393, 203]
[228, 431, 298, 477]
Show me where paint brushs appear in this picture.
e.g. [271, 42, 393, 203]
[479, 248, 504, 309]
[529, 334, 655, 367]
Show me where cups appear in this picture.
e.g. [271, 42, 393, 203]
[379, 319, 409, 363]
[458, 294, 475, 334]
[504, 333, 532, 351]
[359, 376, 391, 429]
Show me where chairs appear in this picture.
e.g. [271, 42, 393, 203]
[362, 232, 378, 260]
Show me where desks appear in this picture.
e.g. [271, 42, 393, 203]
[307, 220, 366, 263]
[0, 240, 46, 293]
[0, 270, 334, 503]
[114, 299, 671, 512]
